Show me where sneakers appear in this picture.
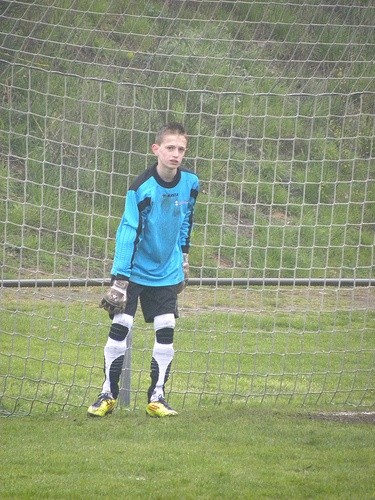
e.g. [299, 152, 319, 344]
[86, 393, 116, 418]
[145, 398, 179, 420]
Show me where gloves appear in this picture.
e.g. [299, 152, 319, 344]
[98, 275, 131, 316]
[176, 256, 192, 296]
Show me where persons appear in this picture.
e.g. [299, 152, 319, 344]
[86, 124, 201, 418]
